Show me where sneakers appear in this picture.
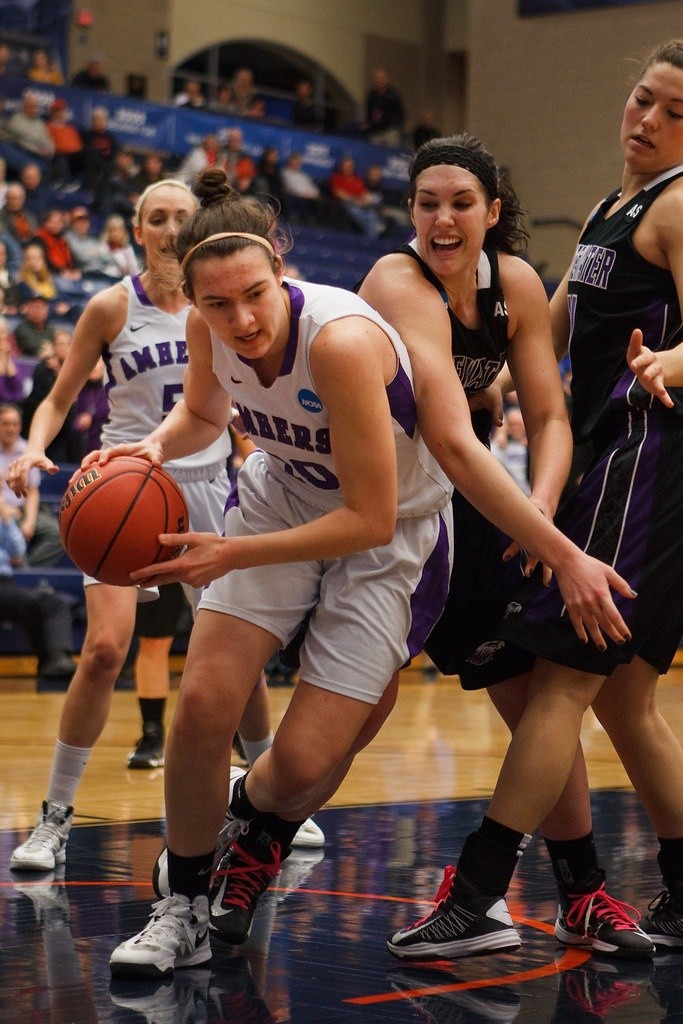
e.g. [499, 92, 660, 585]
[152, 766, 253, 898]
[637, 887, 683, 948]
[387, 864, 522, 957]
[232, 731, 250, 765]
[108, 893, 213, 983]
[386, 967, 520, 1024]
[554, 868, 656, 959]
[552, 946, 653, 1018]
[127, 734, 166, 769]
[9, 800, 74, 871]
[109, 966, 214, 1024]
[204, 955, 275, 1024]
[13, 864, 70, 931]
[289, 817, 325, 848]
[205, 816, 293, 945]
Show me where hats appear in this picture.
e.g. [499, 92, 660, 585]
[70, 207, 86, 221]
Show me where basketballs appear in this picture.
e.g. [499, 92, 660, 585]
[59, 455, 190, 587]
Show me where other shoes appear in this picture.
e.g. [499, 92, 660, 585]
[112, 658, 176, 692]
[36, 658, 79, 692]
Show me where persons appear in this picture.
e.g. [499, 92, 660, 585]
[387, 38, 683, 958]
[6, 178, 325, 871]
[0, 42, 463, 686]
[70, 170, 455, 978]
[194, 135, 656, 960]
